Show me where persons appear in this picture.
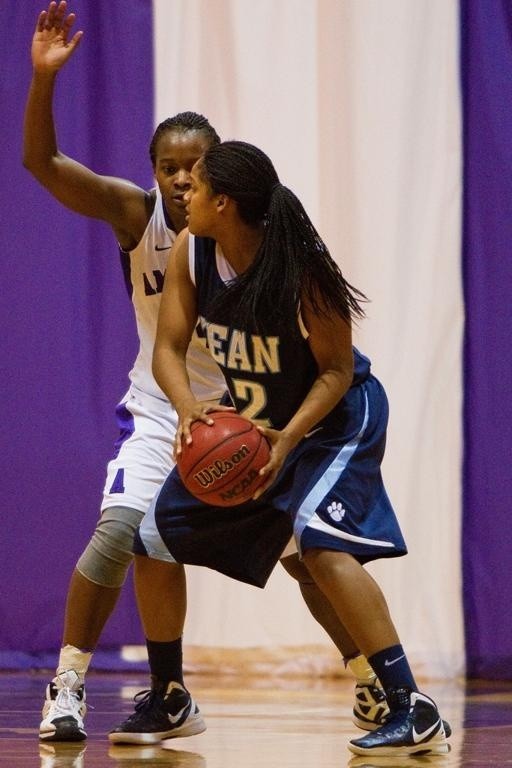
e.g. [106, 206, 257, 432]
[16, 1, 454, 747]
[101, 139, 453, 758]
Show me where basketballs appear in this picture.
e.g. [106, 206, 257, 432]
[177, 411, 271, 508]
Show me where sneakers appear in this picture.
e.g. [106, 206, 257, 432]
[348, 684, 448, 756]
[106, 674, 207, 745]
[37, 668, 89, 741]
[351, 678, 452, 738]
